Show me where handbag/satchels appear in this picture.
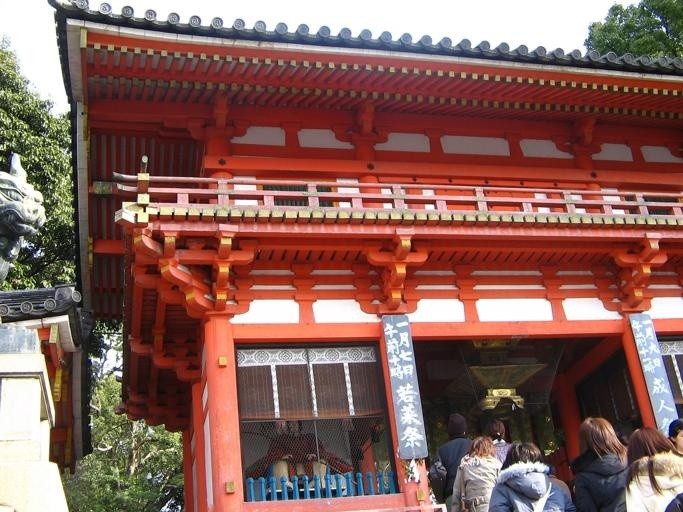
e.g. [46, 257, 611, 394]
[428, 462, 447, 504]
[461, 497, 469, 512]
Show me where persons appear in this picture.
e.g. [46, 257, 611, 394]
[265, 420, 332, 489]
[429, 412, 682, 512]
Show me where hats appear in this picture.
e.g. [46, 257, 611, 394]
[448, 414, 468, 434]
